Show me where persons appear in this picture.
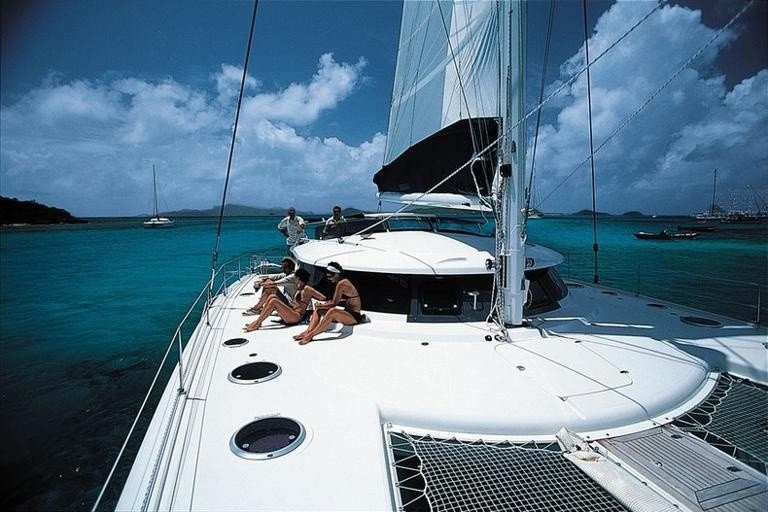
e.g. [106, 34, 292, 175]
[246, 269, 328, 332]
[324, 206, 346, 236]
[294, 262, 363, 344]
[243, 259, 297, 315]
[278, 207, 305, 255]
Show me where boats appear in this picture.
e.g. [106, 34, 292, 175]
[671, 224, 719, 234]
[631, 231, 701, 241]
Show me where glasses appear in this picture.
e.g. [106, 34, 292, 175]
[327, 275, 336, 278]
[333, 211, 342, 213]
[280, 266, 291, 269]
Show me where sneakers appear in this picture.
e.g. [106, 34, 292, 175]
[241, 304, 263, 316]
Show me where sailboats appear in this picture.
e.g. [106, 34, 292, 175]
[89, 1, 767, 512]
[694, 170, 768, 225]
[139, 162, 178, 229]
